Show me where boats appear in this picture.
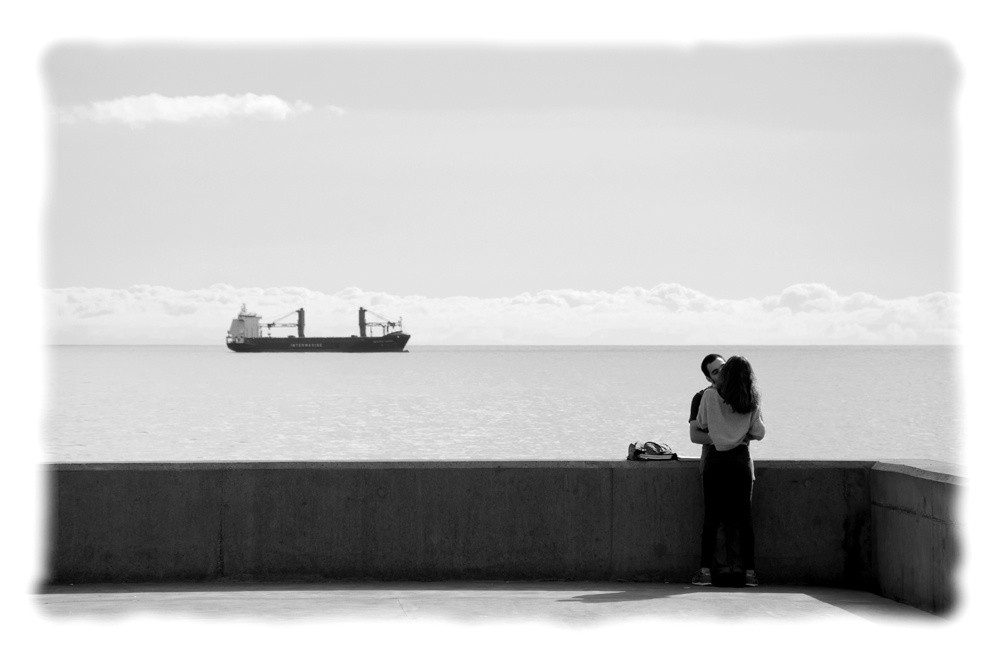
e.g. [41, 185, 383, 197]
[226, 304, 410, 352]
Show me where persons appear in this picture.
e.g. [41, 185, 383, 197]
[688, 354, 766, 587]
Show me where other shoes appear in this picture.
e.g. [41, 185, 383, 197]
[745, 569, 760, 587]
[692, 568, 712, 585]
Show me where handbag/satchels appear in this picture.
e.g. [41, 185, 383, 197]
[627, 440, 678, 460]
